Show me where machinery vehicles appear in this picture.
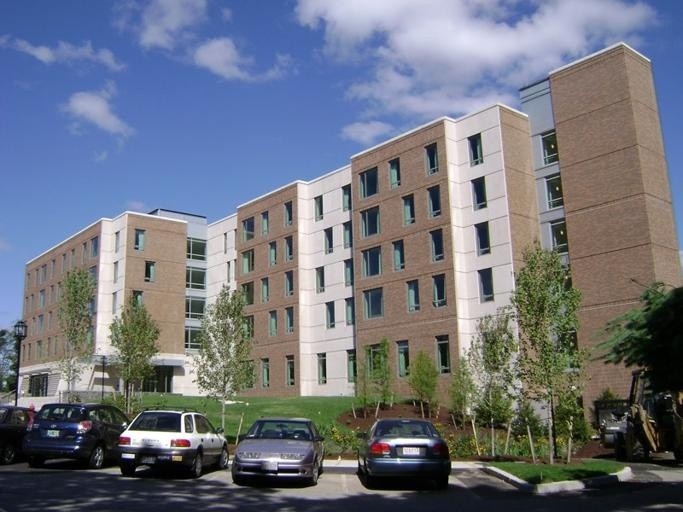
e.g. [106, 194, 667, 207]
[589, 365, 682, 465]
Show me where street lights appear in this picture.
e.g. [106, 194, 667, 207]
[12, 318, 27, 409]
[99, 354, 108, 402]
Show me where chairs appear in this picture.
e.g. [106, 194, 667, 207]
[262, 428, 307, 440]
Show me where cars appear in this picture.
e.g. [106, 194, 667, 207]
[115, 403, 232, 480]
[351, 416, 453, 491]
[230, 414, 328, 488]
[19, 403, 132, 469]
[0, 405, 41, 466]
